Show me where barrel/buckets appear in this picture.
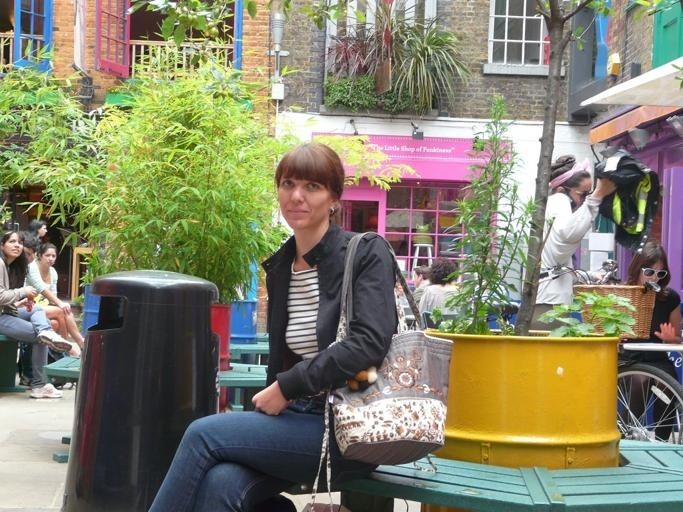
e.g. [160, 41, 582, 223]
[420, 327, 622, 512]
[230, 301, 257, 344]
[82, 283, 101, 337]
[210, 304, 232, 412]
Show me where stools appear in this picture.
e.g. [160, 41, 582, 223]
[411, 243, 434, 277]
[228, 340, 272, 359]
[42, 356, 269, 463]
[70, 246, 94, 302]
[256, 332, 270, 341]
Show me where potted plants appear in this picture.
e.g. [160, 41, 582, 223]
[322, 0, 474, 115]
[0, 40, 422, 407]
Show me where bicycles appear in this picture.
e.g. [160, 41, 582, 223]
[485, 259, 683, 445]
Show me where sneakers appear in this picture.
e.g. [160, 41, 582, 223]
[30, 383, 63, 398]
[36, 330, 72, 353]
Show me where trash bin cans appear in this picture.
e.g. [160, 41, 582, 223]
[61, 270, 220, 511]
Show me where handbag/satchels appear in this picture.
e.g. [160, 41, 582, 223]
[331, 330, 454, 465]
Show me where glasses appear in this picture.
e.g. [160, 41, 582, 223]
[564, 186, 593, 196]
[641, 268, 667, 279]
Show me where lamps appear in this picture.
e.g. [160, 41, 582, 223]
[628, 127, 651, 151]
[600, 144, 619, 159]
[666, 115, 683, 137]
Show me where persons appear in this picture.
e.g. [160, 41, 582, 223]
[146, 141, 396, 512]
[0, 217, 87, 397]
[416, 257, 469, 328]
[412, 265, 434, 304]
[520, 154, 617, 331]
[618, 241, 683, 440]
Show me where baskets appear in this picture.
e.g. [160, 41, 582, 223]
[573, 285, 655, 339]
